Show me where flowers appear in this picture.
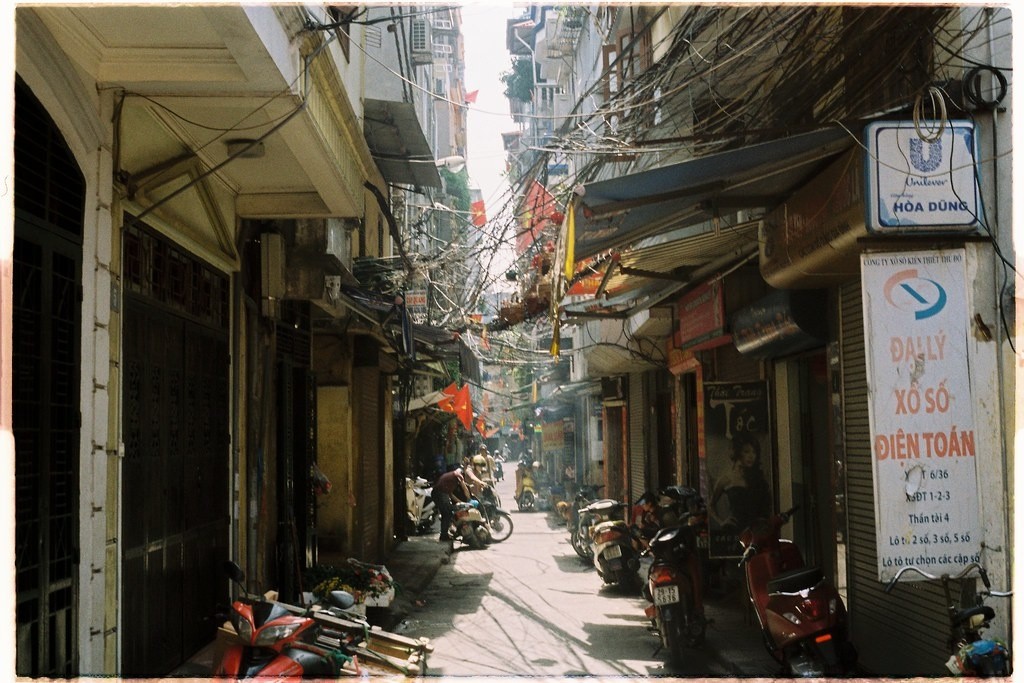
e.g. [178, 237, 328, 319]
[311, 560, 406, 604]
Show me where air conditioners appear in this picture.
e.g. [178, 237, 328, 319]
[409, 17, 431, 53]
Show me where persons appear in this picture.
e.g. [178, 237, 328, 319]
[503, 444, 510, 459]
[525, 450, 533, 470]
[462, 456, 488, 494]
[431, 469, 471, 542]
[492, 450, 506, 480]
[479, 443, 498, 478]
[710, 437, 773, 549]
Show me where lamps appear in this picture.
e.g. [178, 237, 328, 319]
[436, 154, 465, 173]
[225, 138, 266, 158]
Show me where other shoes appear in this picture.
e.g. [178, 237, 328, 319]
[439, 535, 450, 540]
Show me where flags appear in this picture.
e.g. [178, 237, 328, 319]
[469, 199, 486, 227]
[476, 415, 486, 439]
[450, 385, 474, 429]
[516, 180, 558, 252]
[465, 89, 479, 103]
[438, 383, 457, 413]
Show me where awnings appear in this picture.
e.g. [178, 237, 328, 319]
[550, 130, 856, 351]
[390, 322, 483, 384]
[343, 284, 415, 361]
[506, 383, 601, 417]
[366, 97, 443, 196]
[597, 219, 763, 301]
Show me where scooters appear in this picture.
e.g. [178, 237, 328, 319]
[493, 458, 504, 483]
[209, 558, 360, 680]
[513, 469, 536, 513]
[735, 500, 860, 679]
[569, 484, 638, 596]
[635, 508, 708, 670]
[451, 483, 514, 550]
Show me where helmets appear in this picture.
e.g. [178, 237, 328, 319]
[472, 455, 486, 466]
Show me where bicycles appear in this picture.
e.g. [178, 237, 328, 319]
[883, 561, 1012, 678]
